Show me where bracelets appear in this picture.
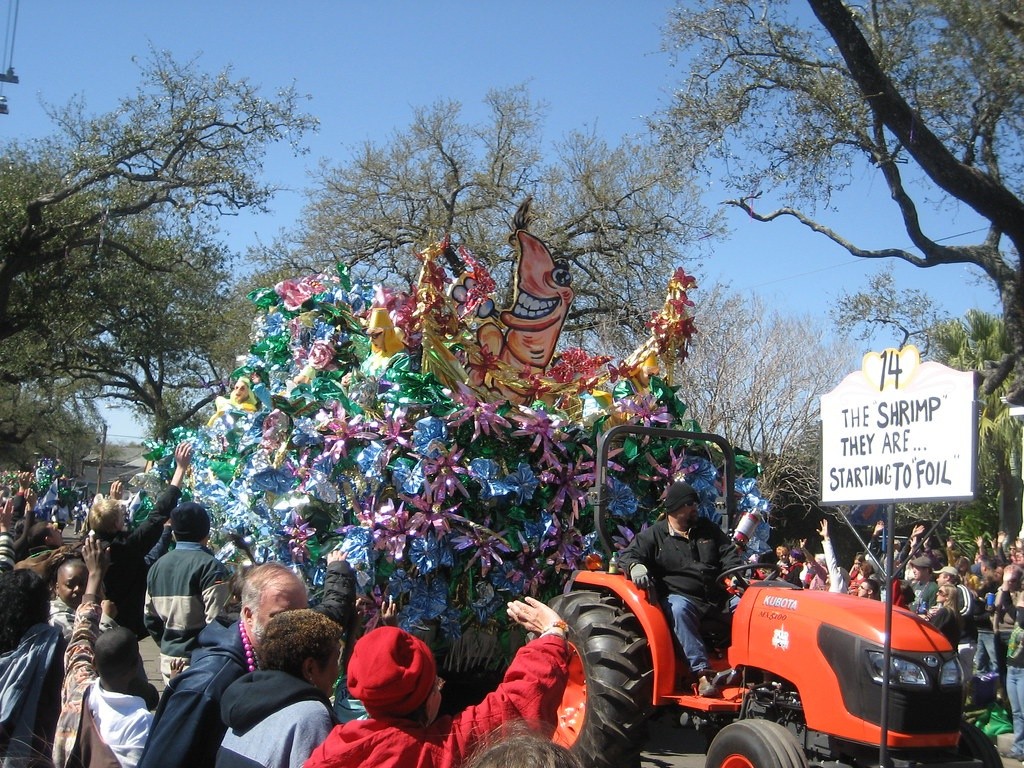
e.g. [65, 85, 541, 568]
[539, 620, 568, 634]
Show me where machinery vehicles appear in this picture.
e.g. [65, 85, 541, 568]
[522, 424, 1004, 768]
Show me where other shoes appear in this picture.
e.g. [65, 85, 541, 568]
[999, 750, 1024, 762]
[695, 670, 719, 695]
[981, 672, 999, 682]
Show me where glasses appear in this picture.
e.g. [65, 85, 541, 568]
[1009, 548, 1016, 551]
[683, 501, 701, 509]
[431, 676, 445, 693]
[937, 590, 947, 597]
[860, 585, 868, 591]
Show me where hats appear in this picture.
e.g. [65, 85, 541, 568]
[170, 501, 211, 542]
[933, 566, 958, 576]
[910, 557, 932, 568]
[790, 549, 802, 560]
[346, 626, 437, 717]
[666, 482, 700, 513]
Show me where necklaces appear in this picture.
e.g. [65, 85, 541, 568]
[252, 646, 263, 670]
[239, 621, 256, 672]
[909, 581, 930, 612]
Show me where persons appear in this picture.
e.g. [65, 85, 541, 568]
[617, 482, 750, 696]
[746, 518, 1024, 761]
[0, 438, 598, 767]
[206, 305, 410, 429]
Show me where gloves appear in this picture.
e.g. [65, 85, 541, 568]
[629, 563, 657, 590]
[730, 576, 751, 592]
